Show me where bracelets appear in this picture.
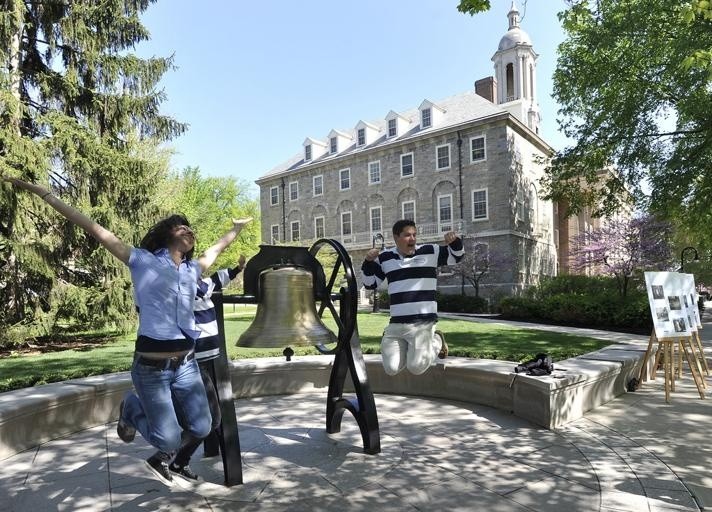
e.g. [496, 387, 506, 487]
[42, 192, 51, 198]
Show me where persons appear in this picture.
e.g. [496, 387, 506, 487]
[143, 254, 248, 487]
[2, 171, 254, 452]
[361, 220, 465, 376]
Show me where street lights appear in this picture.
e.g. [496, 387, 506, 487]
[681, 247, 699, 272]
[373, 232, 386, 313]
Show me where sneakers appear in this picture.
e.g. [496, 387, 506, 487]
[117, 400, 136, 443]
[435, 330, 449, 359]
[169, 458, 203, 485]
[145, 454, 177, 488]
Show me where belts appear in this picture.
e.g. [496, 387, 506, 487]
[134, 350, 194, 369]
